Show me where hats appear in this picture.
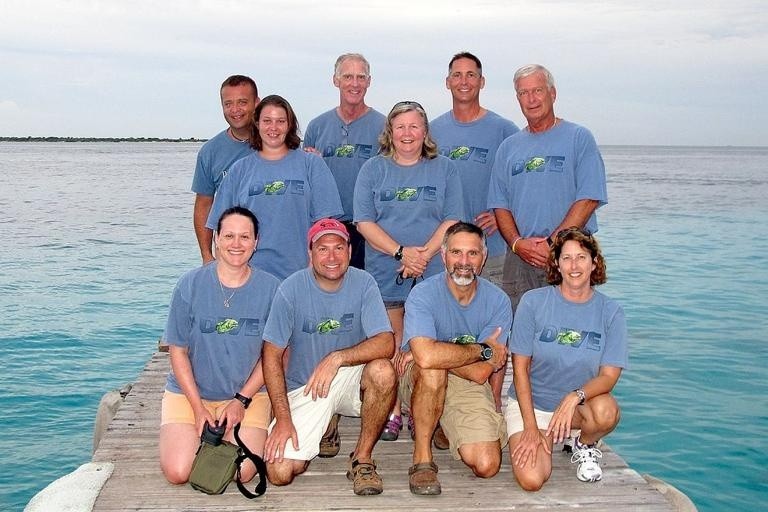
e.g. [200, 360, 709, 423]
[308, 220, 350, 243]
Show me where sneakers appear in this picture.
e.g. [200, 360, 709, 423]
[569, 435, 603, 483]
[317, 413, 341, 459]
[407, 414, 417, 441]
[433, 428, 451, 450]
[564, 434, 576, 451]
[381, 414, 404, 441]
[408, 461, 441, 496]
[345, 452, 383, 496]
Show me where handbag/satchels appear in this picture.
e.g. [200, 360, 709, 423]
[188, 418, 267, 500]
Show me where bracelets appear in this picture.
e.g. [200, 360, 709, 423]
[512, 237, 524, 254]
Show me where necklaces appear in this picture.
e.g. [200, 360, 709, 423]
[217, 259, 248, 307]
[549, 114, 560, 132]
[231, 128, 249, 143]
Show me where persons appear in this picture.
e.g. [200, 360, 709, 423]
[427, 51, 521, 451]
[205, 94, 346, 284]
[352, 101, 463, 440]
[486, 63, 608, 453]
[394, 221, 514, 495]
[504, 225, 628, 491]
[303, 53, 388, 270]
[191, 74, 321, 267]
[160, 206, 282, 485]
[264, 218, 397, 496]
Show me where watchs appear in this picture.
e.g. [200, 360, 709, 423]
[234, 392, 252, 410]
[394, 246, 404, 261]
[546, 236, 559, 251]
[573, 388, 585, 405]
[478, 343, 493, 361]
